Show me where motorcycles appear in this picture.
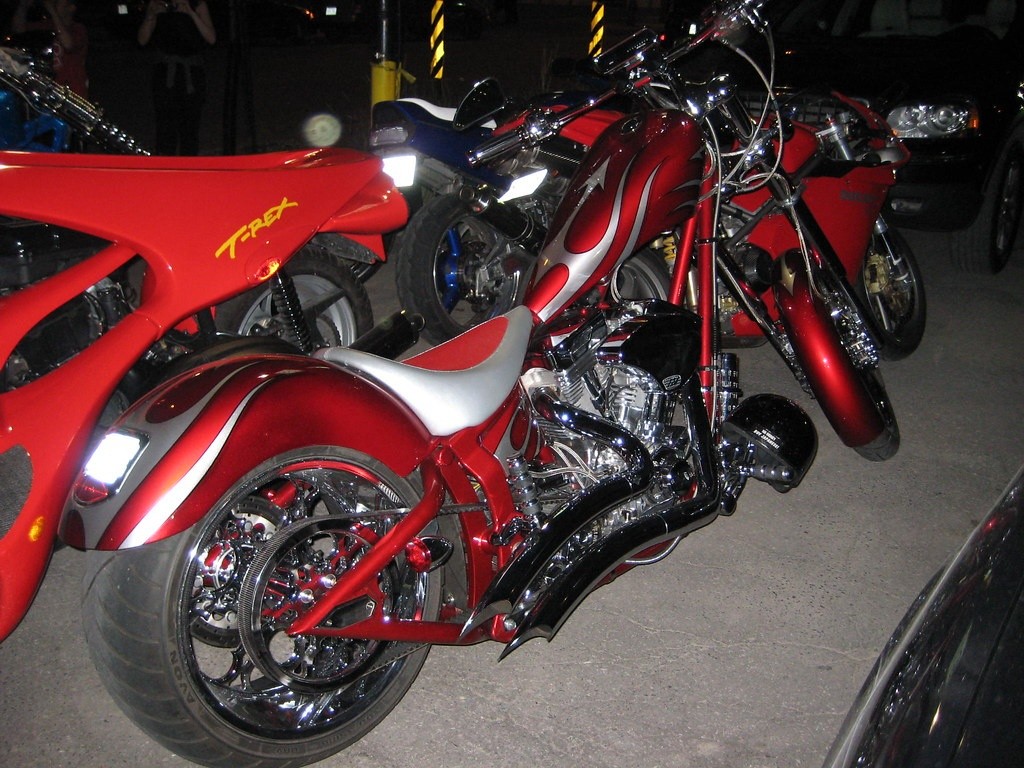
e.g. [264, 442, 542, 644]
[0, 0, 927, 768]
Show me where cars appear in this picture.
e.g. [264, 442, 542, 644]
[718, 0, 1024, 275]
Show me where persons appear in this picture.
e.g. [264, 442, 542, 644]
[137, 0, 216, 159]
[10, 0, 89, 102]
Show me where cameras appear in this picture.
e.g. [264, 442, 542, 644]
[162, 0, 177, 11]
